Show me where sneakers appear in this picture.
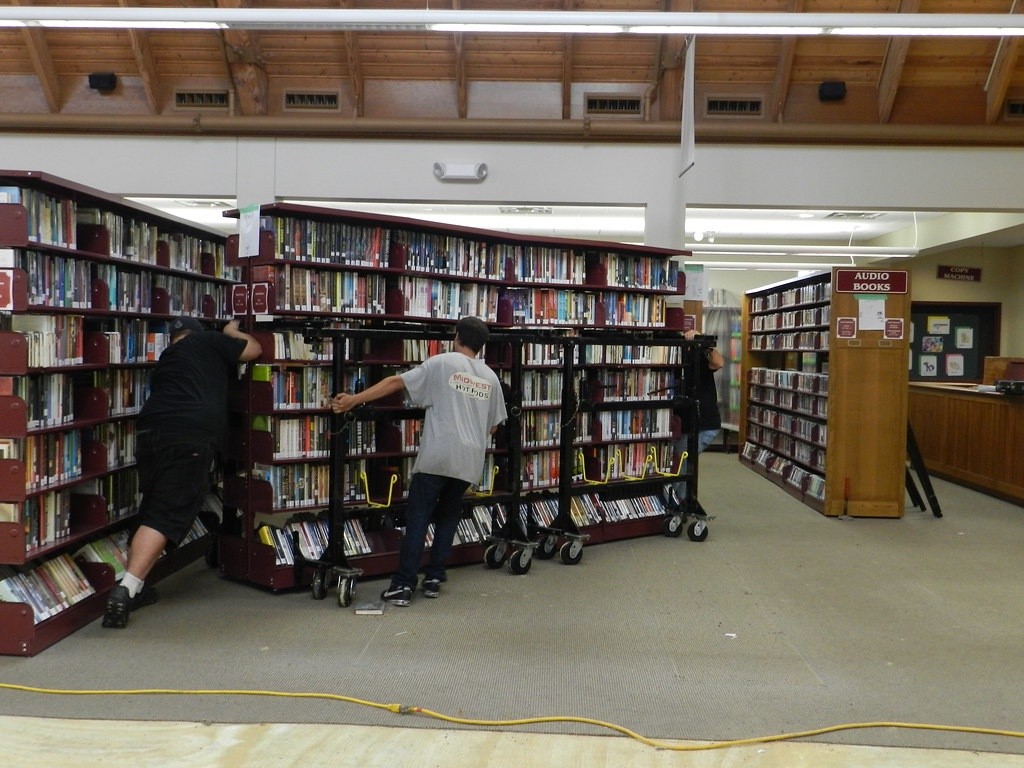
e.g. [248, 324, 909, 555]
[422, 578, 441, 598]
[381, 586, 412, 607]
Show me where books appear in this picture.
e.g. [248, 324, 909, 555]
[748, 283, 832, 350]
[741, 367, 827, 499]
[252, 215, 677, 566]
[0, 186, 240, 624]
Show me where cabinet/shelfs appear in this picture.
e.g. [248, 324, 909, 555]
[738, 267, 913, 518]
[0, 170, 689, 658]
[702, 307, 743, 453]
[908, 381, 1024, 508]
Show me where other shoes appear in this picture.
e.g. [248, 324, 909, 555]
[129, 586, 160, 611]
[101, 585, 131, 629]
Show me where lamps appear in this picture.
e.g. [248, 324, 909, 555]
[684, 211, 920, 271]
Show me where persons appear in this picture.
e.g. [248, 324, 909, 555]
[102, 316, 261, 629]
[674, 330, 724, 504]
[331, 316, 507, 606]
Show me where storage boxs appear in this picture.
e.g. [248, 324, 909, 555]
[983, 356, 1024, 386]
